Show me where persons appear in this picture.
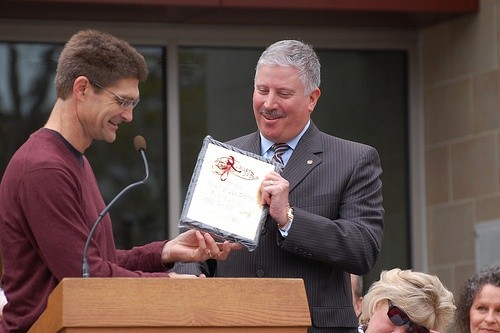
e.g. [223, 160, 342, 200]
[175, 40, 385, 333]
[0, 30, 241, 333]
[357, 268, 457, 333]
[451, 267, 500, 333]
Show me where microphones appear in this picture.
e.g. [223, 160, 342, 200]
[82, 136, 149, 276]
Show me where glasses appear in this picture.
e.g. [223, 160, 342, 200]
[386, 299, 430, 333]
[92, 81, 140, 111]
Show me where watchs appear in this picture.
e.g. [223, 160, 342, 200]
[279, 206, 294, 235]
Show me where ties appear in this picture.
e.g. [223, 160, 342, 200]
[270, 143, 289, 174]
